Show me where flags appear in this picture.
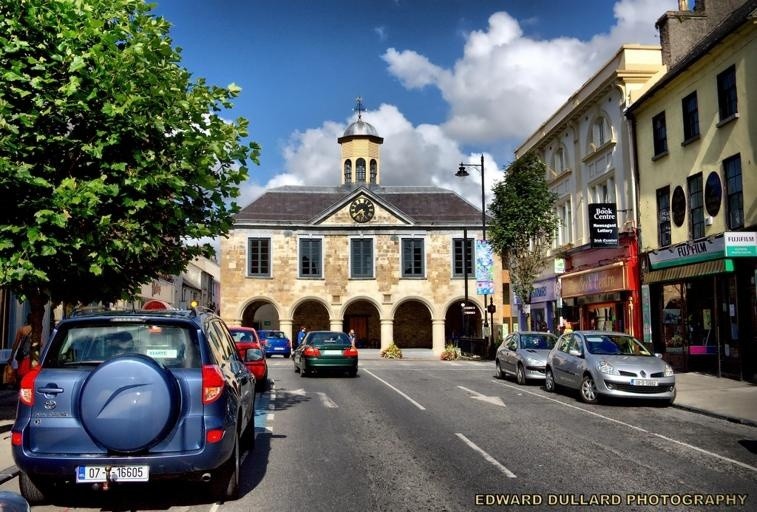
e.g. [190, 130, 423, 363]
[587, 202, 618, 249]
[473, 238, 494, 295]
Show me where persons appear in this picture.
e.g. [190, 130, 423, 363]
[295, 325, 307, 346]
[119, 332, 135, 349]
[347, 328, 356, 344]
[7, 313, 43, 368]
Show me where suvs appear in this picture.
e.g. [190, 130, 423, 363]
[10, 306, 257, 501]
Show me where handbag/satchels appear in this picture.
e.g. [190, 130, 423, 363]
[15, 347, 23, 361]
[3, 363, 16, 385]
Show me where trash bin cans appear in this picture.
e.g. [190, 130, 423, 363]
[471, 339, 487, 360]
[458, 338, 472, 356]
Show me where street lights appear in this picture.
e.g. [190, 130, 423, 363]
[455, 152, 489, 359]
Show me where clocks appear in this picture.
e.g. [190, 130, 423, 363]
[349, 197, 374, 222]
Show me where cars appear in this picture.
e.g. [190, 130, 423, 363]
[495, 331, 576, 386]
[239, 330, 290, 358]
[544, 331, 677, 409]
[226, 326, 267, 389]
[292, 331, 358, 378]
